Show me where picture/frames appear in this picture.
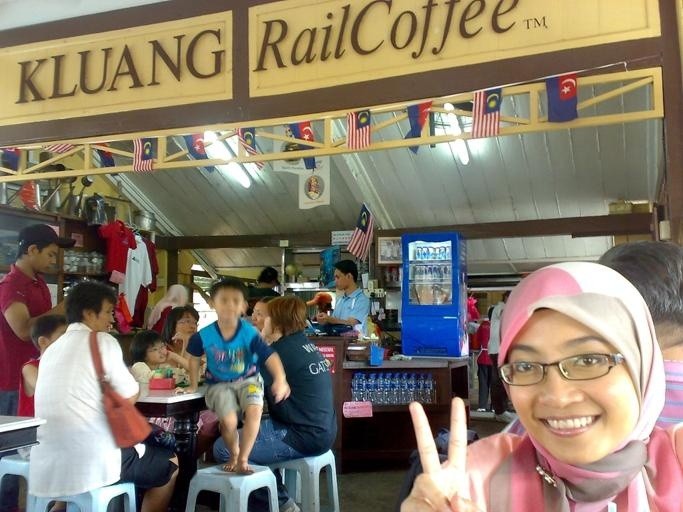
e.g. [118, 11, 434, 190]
[373, 232, 402, 266]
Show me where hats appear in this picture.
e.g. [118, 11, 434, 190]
[18, 223, 76, 249]
[306, 292, 333, 306]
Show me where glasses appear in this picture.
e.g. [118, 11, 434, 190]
[497, 350, 625, 386]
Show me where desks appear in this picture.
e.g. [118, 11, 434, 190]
[342, 358, 469, 475]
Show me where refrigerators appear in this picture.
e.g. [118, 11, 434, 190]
[400, 230, 471, 358]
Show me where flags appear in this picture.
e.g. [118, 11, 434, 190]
[182, 133, 215, 175]
[345, 108, 370, 150]
[93, 143, 118, 178]
[344, 202, 376, 261]
[472, 90, 501, 139]
[595, 239, 682, 434]
[402, 102, 432, 154]
[4, 147, 19, 169]
[544, 76, 581, 124]
[289, 123, 316, 170]
[131, 137, 152, 175]
[235, 128, 264, 173]
[42, 144, 75, 156]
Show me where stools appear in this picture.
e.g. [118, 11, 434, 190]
[0, 454, 139, 511]
[184, 449, 340, 511]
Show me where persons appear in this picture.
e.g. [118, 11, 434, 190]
[248, 294, 278, 334]
[147, 304, 224, 476]
[0, 222, 69, 501]
[12, 311, 67, 462]
[241, 266, 281, 319]
[316, 259, 371, 339]
[142, 282, 189, 336]
[170, 276, 293, 474]
[212, 293, 338, 510]
[473, 304, 496, 413]
[487, 289, 518, 423]
[304, 290, 333, 325]
[126, 328, 194, 451]
[26, 277, 180, 511]
[395, 260, 683, 511]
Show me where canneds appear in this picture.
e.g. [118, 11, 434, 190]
[174, 419, 191, 432]
[416, 247, 451, 260]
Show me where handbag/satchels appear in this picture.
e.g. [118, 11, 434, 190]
[88, 329, 154, 451]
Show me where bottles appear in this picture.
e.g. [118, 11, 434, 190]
[351, 372, 436, 406]
[63, 250, 105, 271]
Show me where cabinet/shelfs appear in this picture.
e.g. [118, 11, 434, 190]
[0, 204, 152, 310]
